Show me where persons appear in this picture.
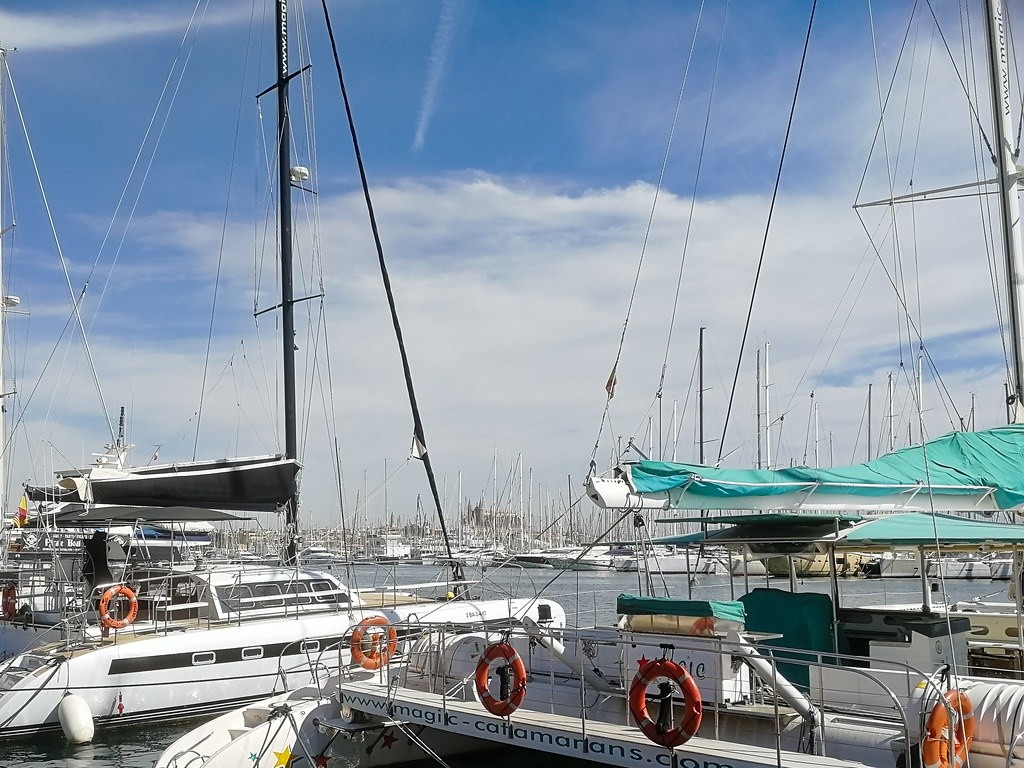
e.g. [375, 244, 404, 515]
[19, 603, 31, 614]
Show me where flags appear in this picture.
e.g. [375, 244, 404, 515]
[605, 364, 617, 399]
[13, 492, 28, 528]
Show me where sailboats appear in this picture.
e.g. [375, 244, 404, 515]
[1, 0, 1024, 767]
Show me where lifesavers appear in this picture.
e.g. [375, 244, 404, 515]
[474, 643, 527, 717]
[99, 585, 139, 629]
[690, 616, 715, 636]
[628, 658, 704, 748]
[350, 615, 398, 671]
[922, 688, 975, 768]
[2, 584, 16, 619]
